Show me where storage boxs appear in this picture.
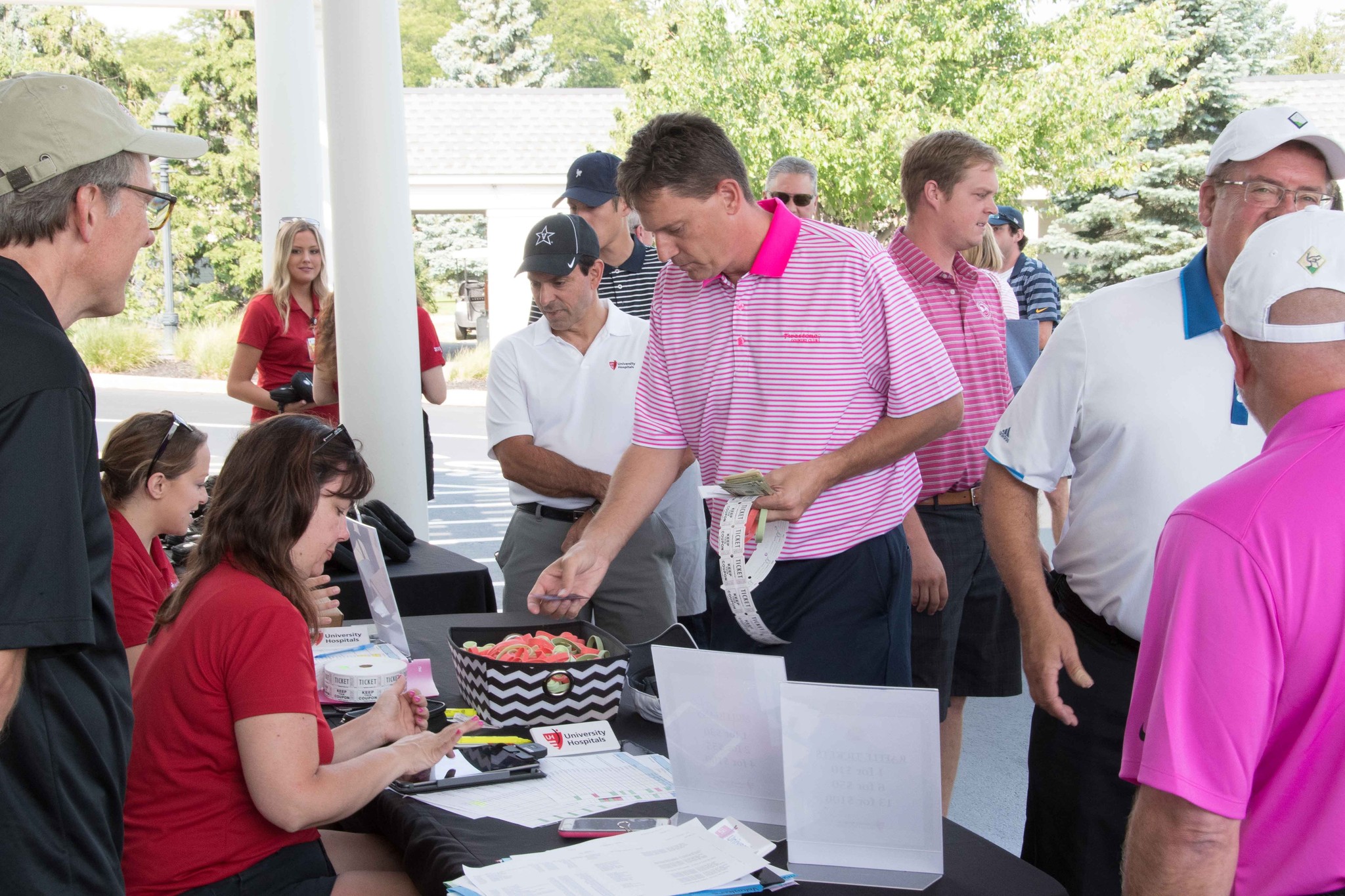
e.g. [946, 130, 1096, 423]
[445, 619, 630, 732]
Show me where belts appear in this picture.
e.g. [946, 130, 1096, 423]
[517, 502, 585, 524]
[914, 485, 982, 506]
[1054, 576, 1141, 649]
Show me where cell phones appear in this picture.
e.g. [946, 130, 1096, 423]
[558, 818, 669, 838]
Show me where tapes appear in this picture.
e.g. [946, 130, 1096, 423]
[326, 657, 407, 704]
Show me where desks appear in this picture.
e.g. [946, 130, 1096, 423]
[162, 507, 499, 622]
[309, 620, 1074, 896]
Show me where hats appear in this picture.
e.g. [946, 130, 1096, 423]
[1205, 106, 1345, 180]
[514, 213, 600, 279]
[0, 71, 209, 197]
[987, 205, 1024, 232]
[552, 150, 625, 208]
[1223, 205, 1345, 343]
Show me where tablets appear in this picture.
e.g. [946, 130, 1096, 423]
[393, 743, 539, 788]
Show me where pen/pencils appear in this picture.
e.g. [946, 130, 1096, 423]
[315, 644, 374, 659]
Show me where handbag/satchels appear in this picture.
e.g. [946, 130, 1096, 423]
[1005, 319, 1039, 396]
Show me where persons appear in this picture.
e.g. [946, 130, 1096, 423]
[528, 151, 710, 618]
[0, 74, 210, 896]
[977, 103, 1345, 896]
[627, 209, 655, 248]
[528, 113, 963, 688]
[1117, 204, 1344, 894]
[884, 129, 1024, 817]
[958, 205, 1071, 543]
[486, 213, 677, 645]
[99, 410, 210, 685]
[227, 216, 339, 428]
[120, 412, 484, 896]
[312, 292, 446, 501]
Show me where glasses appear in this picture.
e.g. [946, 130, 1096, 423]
[311, 423, 356, 455]
[1215, 179, 1337, 212]
[278, 217, 320, 233]
[72, 180, 177, 230]
[765, 190, 817, 207]
[634, 225, 654, 238]
[146, 410, 193, 489]
